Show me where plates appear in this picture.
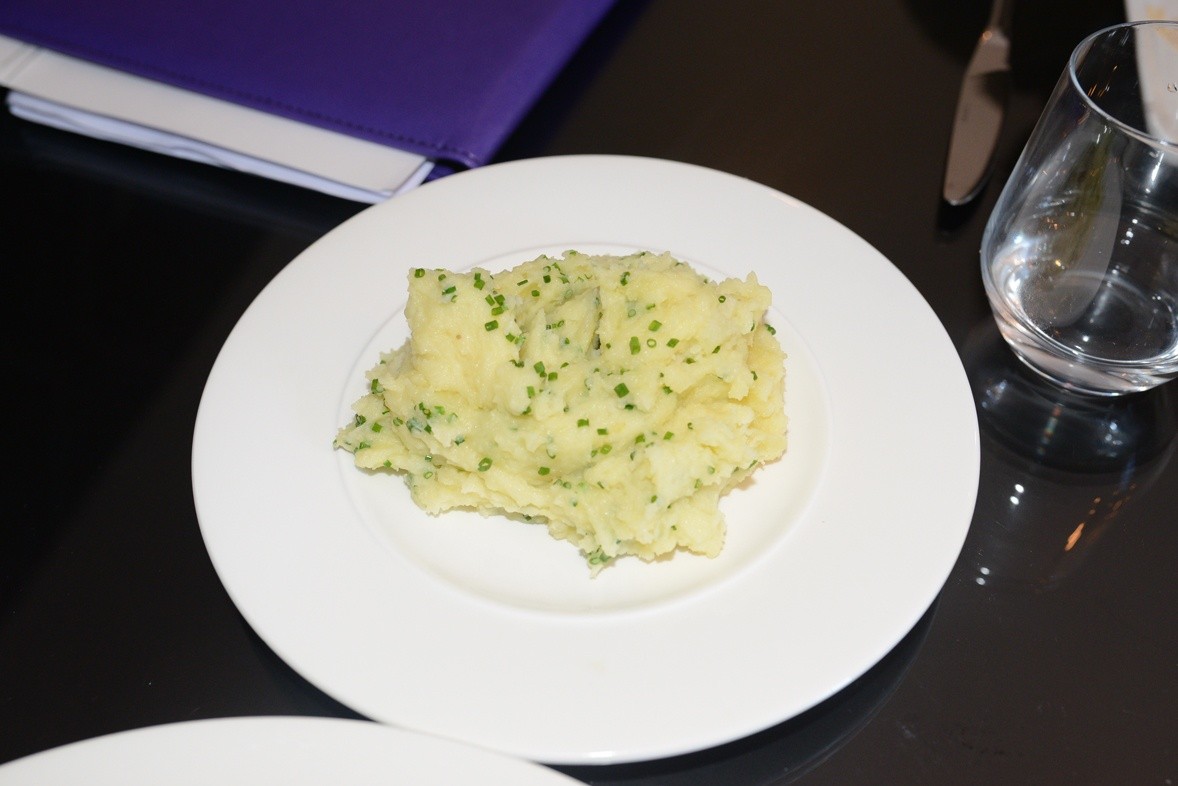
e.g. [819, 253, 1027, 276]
[190, 148, 979, 768]
[5, 719, 590, 786]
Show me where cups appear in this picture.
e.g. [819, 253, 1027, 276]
[979, 16, 1176, 398]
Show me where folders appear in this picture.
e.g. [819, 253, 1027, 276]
[0, 0, 626, 205]
